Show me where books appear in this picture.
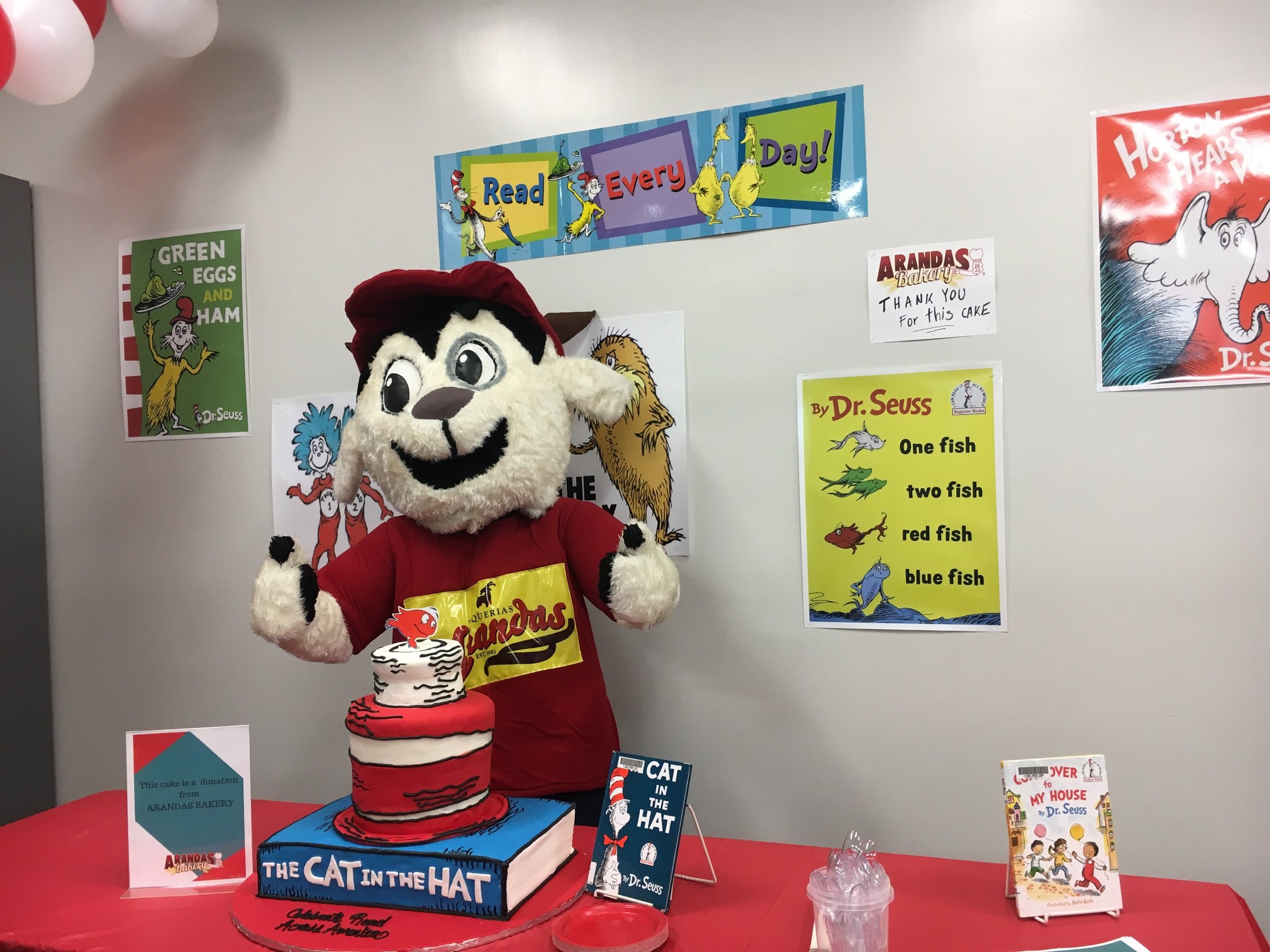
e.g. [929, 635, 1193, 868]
[253, 791, 577, 923]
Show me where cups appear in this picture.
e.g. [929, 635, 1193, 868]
[806, 866, 895, 952]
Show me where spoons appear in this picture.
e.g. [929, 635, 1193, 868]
[809, 829, 886, 952]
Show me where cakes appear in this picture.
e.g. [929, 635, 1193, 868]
[332, 606, 511, 847]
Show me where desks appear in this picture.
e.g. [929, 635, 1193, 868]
[0, 788, 1270, 952]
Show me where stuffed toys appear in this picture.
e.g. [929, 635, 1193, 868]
[250, 260, 680, 826]
